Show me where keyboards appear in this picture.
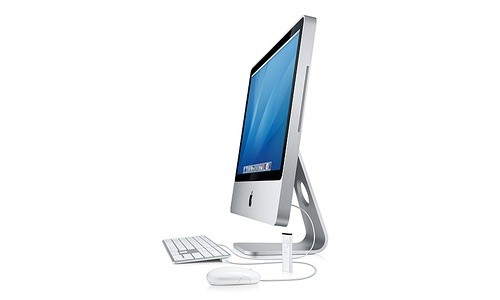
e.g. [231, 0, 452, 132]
[162, 234, 229, 265]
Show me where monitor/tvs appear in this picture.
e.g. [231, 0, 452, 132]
[230, 15, 329, 257]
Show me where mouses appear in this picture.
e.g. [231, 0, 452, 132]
[206, 265, 260, 285]
